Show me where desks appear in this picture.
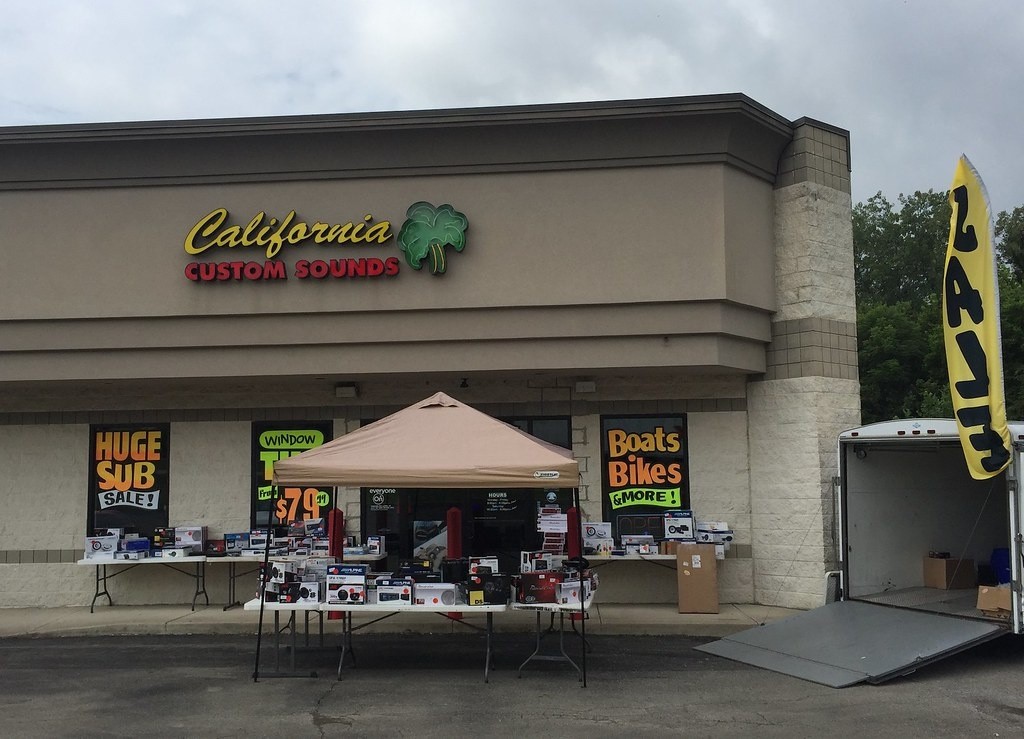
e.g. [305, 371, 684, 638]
[511, 590, 595, 681]
[550, 554, 726, 562]
[77, 555, 209, 612]
[243, 598, 507, 683]
[206, 552, 388, 611]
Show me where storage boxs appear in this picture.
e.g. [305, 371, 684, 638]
[924, 556, 976, 589]
[976, 585, 1012, 619]
[676, 543, 719, 614]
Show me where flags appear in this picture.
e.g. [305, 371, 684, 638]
[939, 157, 1014, 482]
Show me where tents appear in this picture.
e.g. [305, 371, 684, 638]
[252, 390, 589, 686]
